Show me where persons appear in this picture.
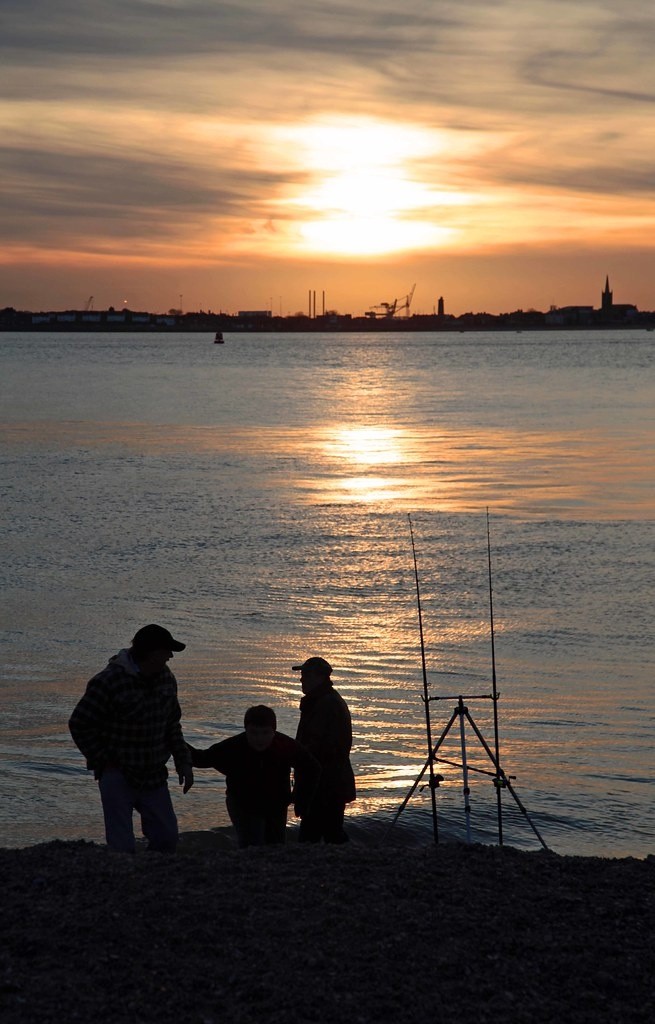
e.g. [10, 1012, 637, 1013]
[289, 657, 357, 842]
[67, 624, 194, 848]
[186, 705, 297, 833]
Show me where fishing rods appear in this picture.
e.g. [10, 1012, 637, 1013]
[486, 505, 517, 846]
[407, 511, 444, 844]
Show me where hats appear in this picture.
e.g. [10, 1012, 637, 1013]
[292, 657, 332, 676]
[134, 624, 186, 652]
